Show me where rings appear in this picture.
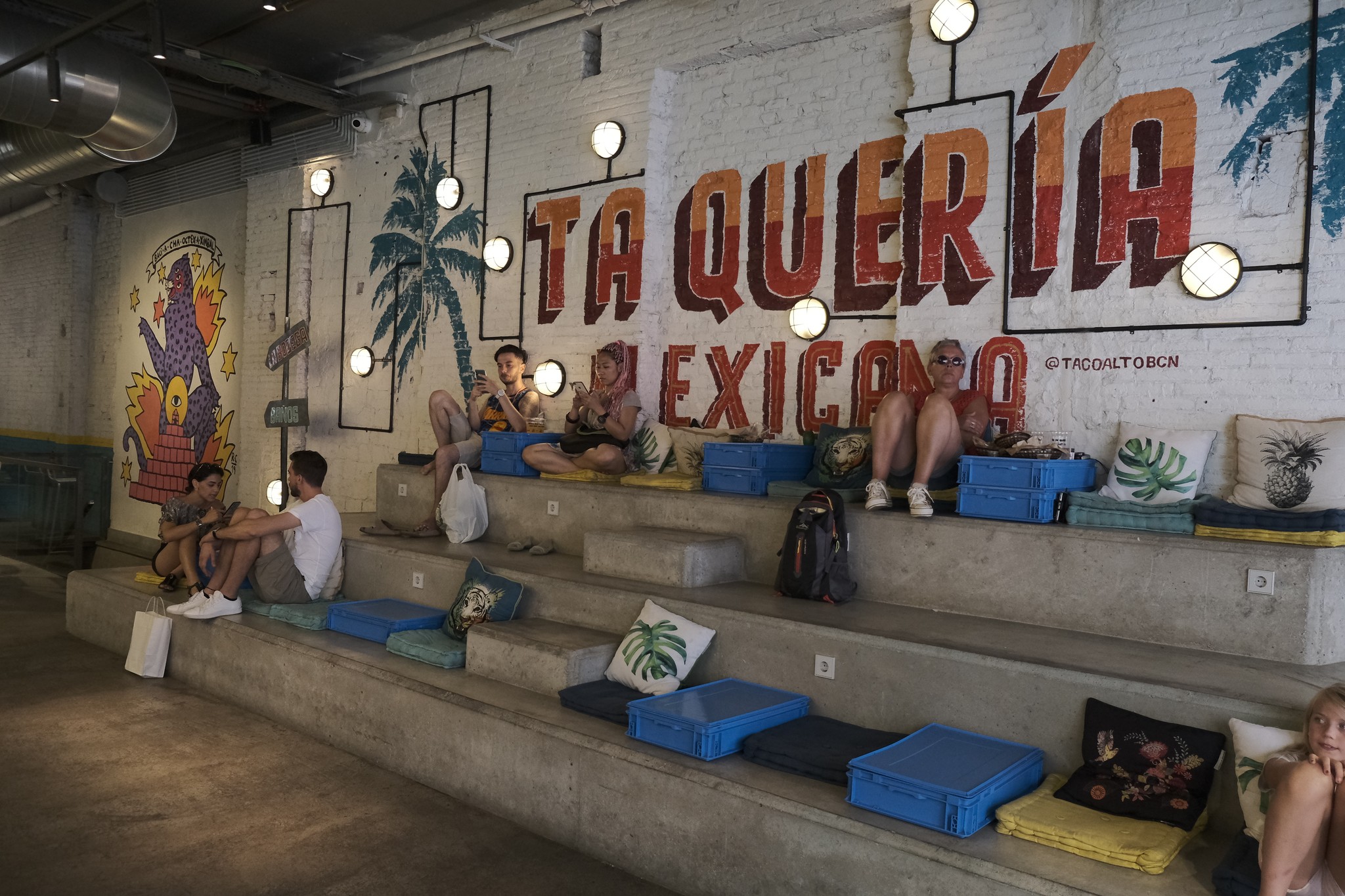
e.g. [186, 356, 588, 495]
[971, 421, 974, 424]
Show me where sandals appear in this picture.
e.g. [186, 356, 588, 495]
[187, 580, 206, 598]
[158, 573, 178, 591]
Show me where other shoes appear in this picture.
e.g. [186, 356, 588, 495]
[507, 536, 532, 551]
[529, 539, 555, 555]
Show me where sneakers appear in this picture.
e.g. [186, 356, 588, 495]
[907, 482, 934, 517]
[166, 589, 209, 615]
[183, 590, 242, 619]
[865, 478, 893, 511]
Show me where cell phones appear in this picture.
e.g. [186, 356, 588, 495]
[573, 381, 590, 396]
[222, 502, 242, 518]
[475, 369, 490, 393]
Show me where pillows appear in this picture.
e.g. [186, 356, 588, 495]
[1051, 698, 1311, 871]
[1097, 414, 1345, 511]
[606, 599, 716, 695]
[808, 424, 874, 489]
[621, 416, 756, 474]
[441, 556, 524, 643]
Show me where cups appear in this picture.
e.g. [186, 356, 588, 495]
[525, 417, 547, 433]
[1028, 431, 1072, 449]
[748, 421, 769, 441]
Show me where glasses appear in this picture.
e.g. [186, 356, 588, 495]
[931, 355, 965, 368]
[193, 462, 221, 477]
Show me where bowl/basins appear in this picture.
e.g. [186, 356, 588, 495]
[1035, 453, 1052, 460]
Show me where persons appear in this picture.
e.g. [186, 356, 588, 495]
[151, 463, 232, 597]
[412, 344, 539, 532]
[166, 450, 342, 619]
[865, 339, 989, 517]
[1258, 683, 1345, 896]
[522, 340, 641, 475]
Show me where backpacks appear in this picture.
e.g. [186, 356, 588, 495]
[773, 488, 857, 605]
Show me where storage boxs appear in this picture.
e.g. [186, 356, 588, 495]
[327, 597, 448, 646]
[479, 431, 565, 477]
[701, 441, 816, 496]
[846, 723, 1045, 836]
[627, 678, 809, 760]
[956, 453, 1097, 523]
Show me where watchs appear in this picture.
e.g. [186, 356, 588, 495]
[597, 412, 610, 424]
[195, 519, 203, 528]
[212, 527, 221, 540]
[495, 388, 505, 399]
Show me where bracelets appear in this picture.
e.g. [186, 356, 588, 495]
[566, 411, 580, 424]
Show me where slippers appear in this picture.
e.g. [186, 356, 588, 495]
[401, 528, 439, 538]
[359, 519, 403, 536]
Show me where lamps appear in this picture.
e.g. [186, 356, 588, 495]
[929, 0, 979, 100]
[266, 479, 282, 506]
[1180, 242, 1303, 301]
[310, 168, 334, 206]
[436, 98, 628, 272]
[523, 359, 566, 398]
[790, 297, 897, 340]
[350, 346, 391, 378]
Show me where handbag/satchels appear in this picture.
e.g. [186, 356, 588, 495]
[124, 596, 173, 677]
[559, 420, 630, 454]
[435, 464, 489, 543]
